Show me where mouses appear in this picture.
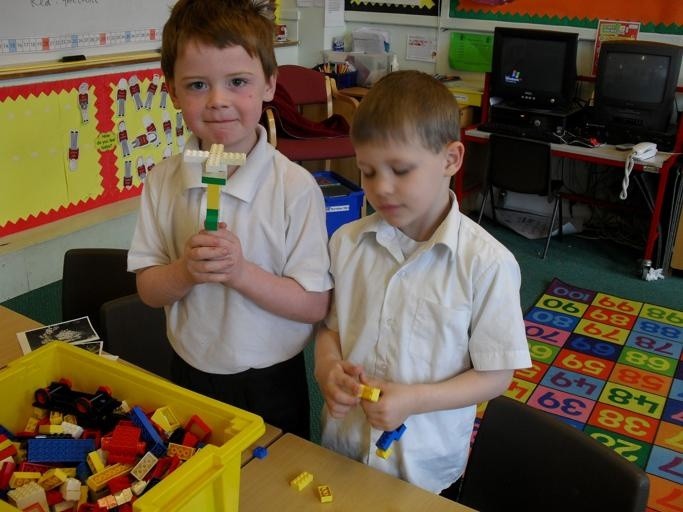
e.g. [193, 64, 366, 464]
[567, 136, 592, 147]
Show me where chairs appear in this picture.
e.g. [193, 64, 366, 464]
[457, 395, 650, 511]
[62, 249, 138, 352]
[476, 133, 564, 260]
[258, 63, 366, 220]
[101, 295, 180, 385]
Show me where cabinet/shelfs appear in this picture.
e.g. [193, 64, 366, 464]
[298, 80, 474, 189]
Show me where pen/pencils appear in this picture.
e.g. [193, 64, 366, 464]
[318, 62, 349, 75]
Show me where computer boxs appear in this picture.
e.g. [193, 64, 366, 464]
[491, 98, 584, 136]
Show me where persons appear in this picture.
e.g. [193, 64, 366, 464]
[126, 1, 333, 442]
[315, 68, 533, 498]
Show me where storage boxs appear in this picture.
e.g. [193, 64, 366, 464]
[0, 341, 267, 512]
[310, 171, 364, 239]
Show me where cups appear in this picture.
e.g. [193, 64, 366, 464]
[276, 24, 288, 42]
[333, 37, 344, 52]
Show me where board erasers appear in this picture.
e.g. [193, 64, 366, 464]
[62, 54, 86, 62]
[0, 0, 179, 80]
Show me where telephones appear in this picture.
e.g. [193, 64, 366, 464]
[627, 141, 658, 161]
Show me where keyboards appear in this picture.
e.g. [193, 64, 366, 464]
[478, 121, 562, 144]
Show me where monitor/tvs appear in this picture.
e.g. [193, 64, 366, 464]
[490, 27, 579, 109]
[593, 39, 683, 132]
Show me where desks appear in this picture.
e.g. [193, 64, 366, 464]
[239, 432, 478, 512]
[1, 305, 283, 469]
[454, 70, 681, 280]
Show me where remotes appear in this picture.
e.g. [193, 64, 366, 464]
[615, 143, 635, 150]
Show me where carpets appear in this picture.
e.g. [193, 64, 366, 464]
[471, 278, 683, 512]
